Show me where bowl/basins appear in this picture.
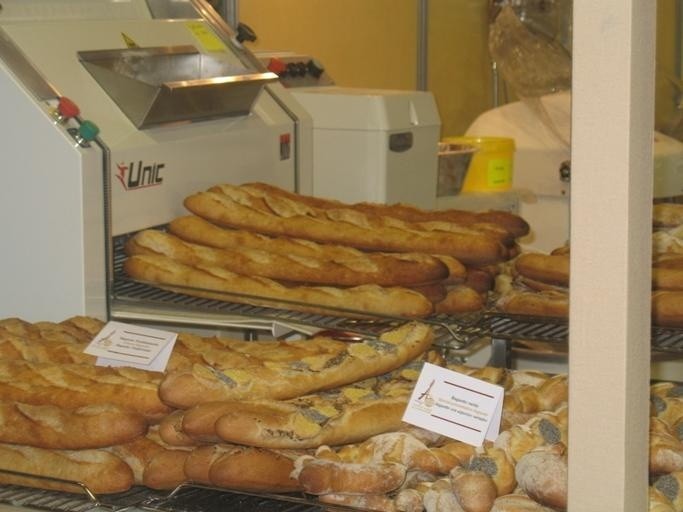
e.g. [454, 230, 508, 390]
[436, 144, 480, 198]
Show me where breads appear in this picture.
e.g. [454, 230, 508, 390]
[125, 183, 530, 319]
[495, 203, 683, 326]
[1, 320, 683, 512]
